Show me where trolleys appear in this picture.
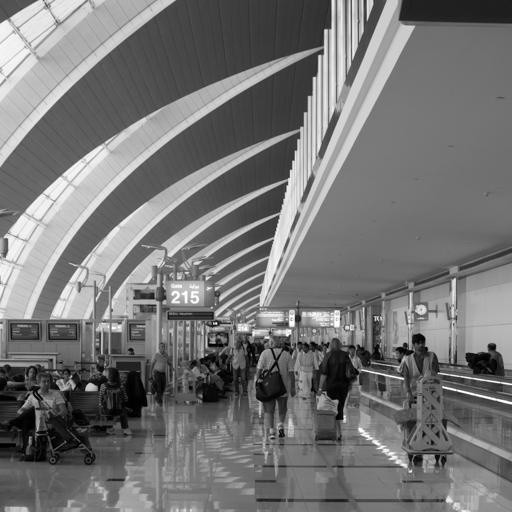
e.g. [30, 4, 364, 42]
[396, 368, 456, 465]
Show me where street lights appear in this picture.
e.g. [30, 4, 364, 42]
[137, 238, 222, 397]
[60, 258, 99, 363]
[92, 267, 117, 355]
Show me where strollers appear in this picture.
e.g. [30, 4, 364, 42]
[29, 385, 100, 465]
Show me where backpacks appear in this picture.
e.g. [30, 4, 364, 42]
[104, 383, 123, 411]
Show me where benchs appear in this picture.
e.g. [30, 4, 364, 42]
[186, 353, 223, 401]
[0, 380, 128, 454]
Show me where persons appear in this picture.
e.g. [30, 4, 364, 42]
[400, 333, 441, 462]
[488, 343, 506, 376]
[150, 338, 414, 445]
[1, 347, 136, 462]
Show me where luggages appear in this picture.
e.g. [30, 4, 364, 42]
[314, 391, 337, 441]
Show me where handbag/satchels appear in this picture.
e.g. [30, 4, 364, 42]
[346, 360, 359, 378]
[317, 393, 339, 415]
[35, 407, 51, 436]
[200, 383, 218, 402]
[256, 371, 286, 401]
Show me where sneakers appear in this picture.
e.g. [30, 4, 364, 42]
[1, 421, 12, 432]
[106, 428, 115, 435]
[279, 425, 285, 438]
[124, 429, 133, 436]
[270, 433, 275, 439]
